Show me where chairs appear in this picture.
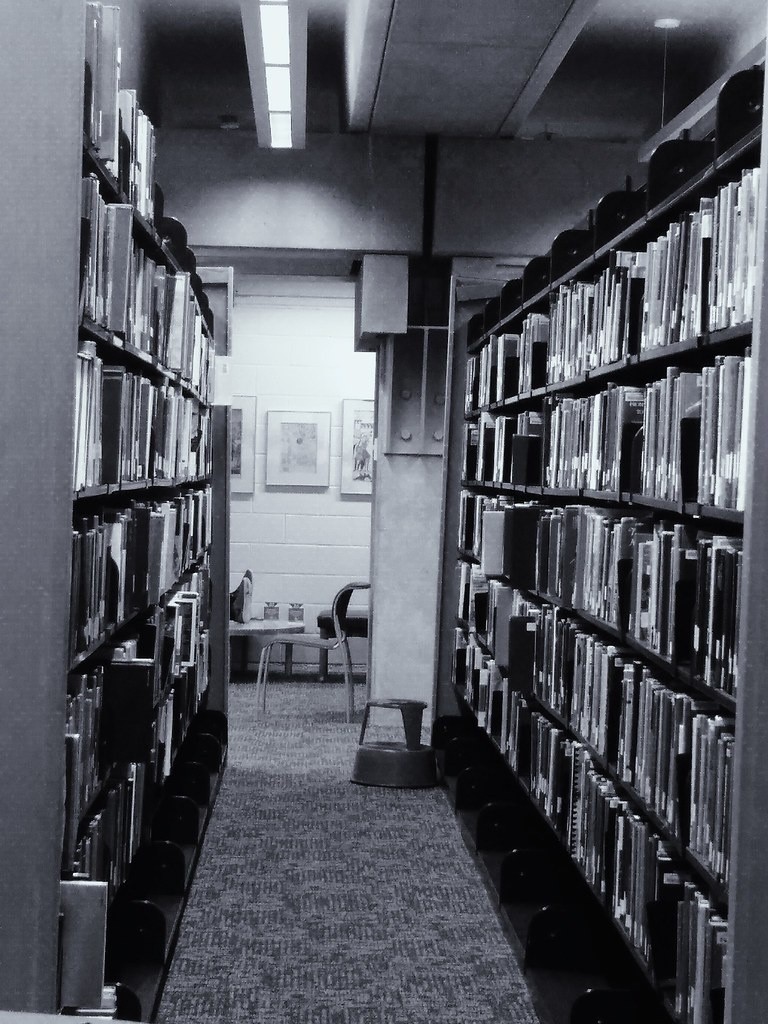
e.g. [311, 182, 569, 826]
[256, 582, 372, 724]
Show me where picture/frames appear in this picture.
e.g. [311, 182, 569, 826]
[231, 394, 257, 493]
[266, 411, 330, 486]
[340, 398, 374, 494]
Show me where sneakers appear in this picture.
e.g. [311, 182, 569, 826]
[233, 569, 254, 624]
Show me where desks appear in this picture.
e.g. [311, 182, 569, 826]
[229, 619, 305, 676]
[317, 611, 369, 681]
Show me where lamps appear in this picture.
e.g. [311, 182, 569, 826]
[636, 18, 767, 162]
[241, 0, 309, 148]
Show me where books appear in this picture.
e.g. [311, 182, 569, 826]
[59, 4, 229, 973]
[444, 164, 768, 1009]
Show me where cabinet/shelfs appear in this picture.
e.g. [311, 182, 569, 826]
[431, 120, 768, 1024]
[0, 1, 233, 1018]
